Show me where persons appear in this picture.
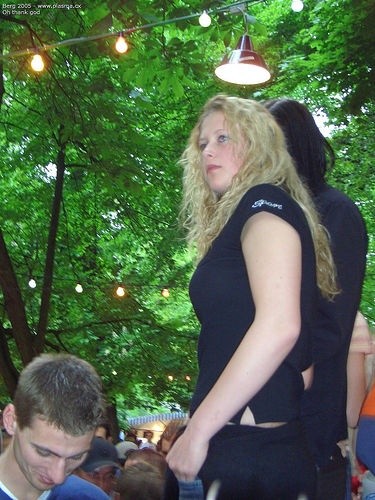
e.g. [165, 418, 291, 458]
[344, 308, 375, 500]
[0, 353, 208, 500]
[265, 99, 370, 500]
[159, 96, 341, 500]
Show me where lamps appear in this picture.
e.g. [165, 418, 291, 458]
[215, 35, 271, 86]
